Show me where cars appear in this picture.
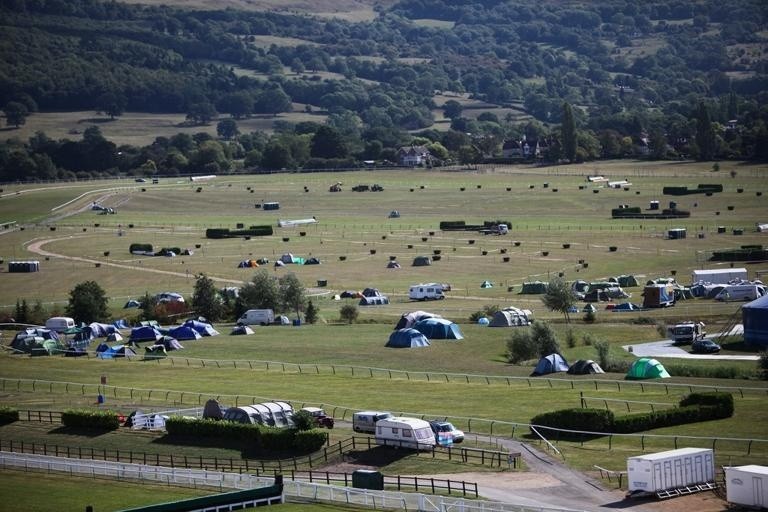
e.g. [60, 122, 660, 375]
[134, 178, 146, 182]
[690, 338, 720, 353]
[90, 204, 104, 210]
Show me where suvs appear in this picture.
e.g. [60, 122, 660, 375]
[426, 418, 465, 444]
[298, 405, 335, 430]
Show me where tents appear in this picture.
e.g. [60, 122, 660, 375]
[143, 413, 168, 428]
[519, 267, 767, 312]
[9, 314, 220, 360]
[201, 398, 227, 420]
[228, 325, 255, 335]
[274, 314, 290, 325]
[387, 261, 401, 268]
[384, 326, 430, 348]
[154, 291, 185, 305]
[534, 351, 569, 375]
[363, 287, 381, 297]
[341, 290, 353, 298]
[238, 252, 322, 267]
[123, 409, 148, 427]
[412, 317, 465, 340]
[740, 292, 768, 349]
[478, 317, 489, 327]
[480, 280, 494, 289]
[487, 307, 535, 327]
[412, 255, 431, 266]
[393, 311, 442, 330]
[122, 300, 140, 308]
[180, 248, 195, 256]
[624, 358, 671, 379]
[224, 400, 295, 430]
[358, 296, 389, 305]
[352, 291, 365, 298]
[567, 358, 606, 374]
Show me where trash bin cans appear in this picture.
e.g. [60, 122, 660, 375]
[352, 470, 385, 491]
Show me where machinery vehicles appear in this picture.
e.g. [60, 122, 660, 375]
[371, 184, 384, 192]
[329, 181, 343, 192]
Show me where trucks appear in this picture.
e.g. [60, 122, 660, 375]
[477, 224, 509, 235]
[374, 415, 436, 452]
[235, 308, 275, 327]
[669, 321, 706, 345]
[713, 284, 758, 301]
[350, 411, 395, 434]
[407, 285, 446, 301]
